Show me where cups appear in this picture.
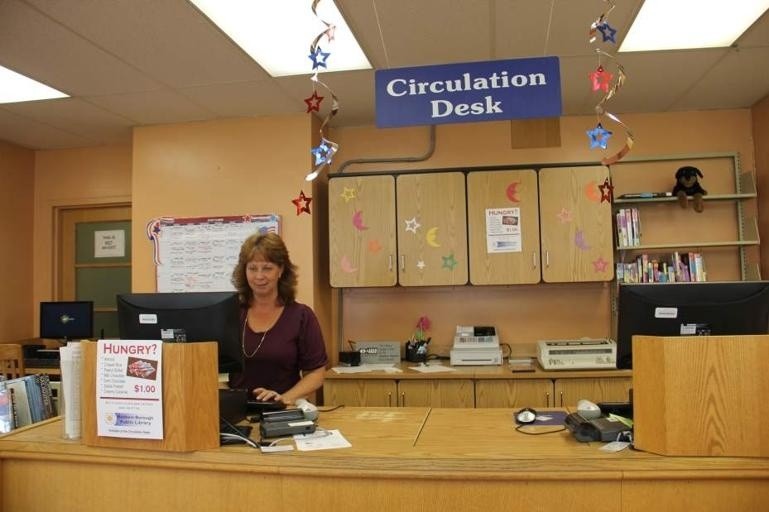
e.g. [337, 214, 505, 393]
[406, 340, 428, 362]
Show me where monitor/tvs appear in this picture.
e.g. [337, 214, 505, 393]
[117, 291, 241, 375]
[37, 300, 94, 352]
[616, 280, 769, 369]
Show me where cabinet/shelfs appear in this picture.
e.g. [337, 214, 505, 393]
[475, 380, 633, 407]
[467, 164, 616, 284]
[322, 379, 474, 407]
[327, 172, 469, 289]
[609, 155, 760, 283]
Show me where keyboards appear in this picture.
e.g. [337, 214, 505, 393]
[247, 399, 287, 412]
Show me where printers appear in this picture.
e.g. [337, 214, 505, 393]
[535, 336, 618, 371]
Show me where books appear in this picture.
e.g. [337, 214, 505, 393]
[0, 374, 54, 433]
[620, 250, 705, 283]
[615, 206, 640, 245]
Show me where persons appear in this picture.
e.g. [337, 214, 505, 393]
[225, 231, 328, 407]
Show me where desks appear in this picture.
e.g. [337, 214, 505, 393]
[37, 348, 63, 354]
[1, 405, 768, 512]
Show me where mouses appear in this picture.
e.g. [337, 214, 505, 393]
[516, 407, 537, 423]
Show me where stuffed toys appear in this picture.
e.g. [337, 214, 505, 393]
[673, 166, 707, 212]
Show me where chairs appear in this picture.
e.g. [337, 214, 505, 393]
[0, 343, 24, 380]
[49, 382, 60, 416]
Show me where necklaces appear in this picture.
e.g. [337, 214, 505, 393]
[242, 305, 276, 357]
